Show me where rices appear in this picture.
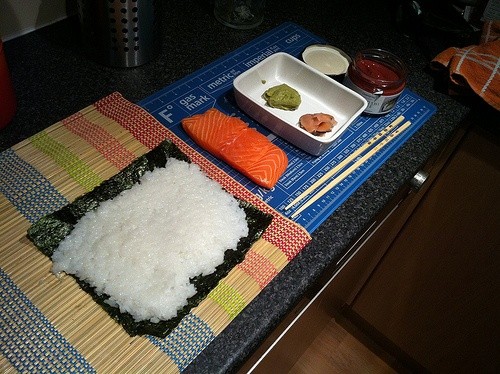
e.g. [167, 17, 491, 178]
[49, 157, 249, 324]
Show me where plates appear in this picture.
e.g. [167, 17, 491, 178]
[232, 51, 368, 157]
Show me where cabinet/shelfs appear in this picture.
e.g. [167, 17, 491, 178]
[233, 109, 499, 374]
